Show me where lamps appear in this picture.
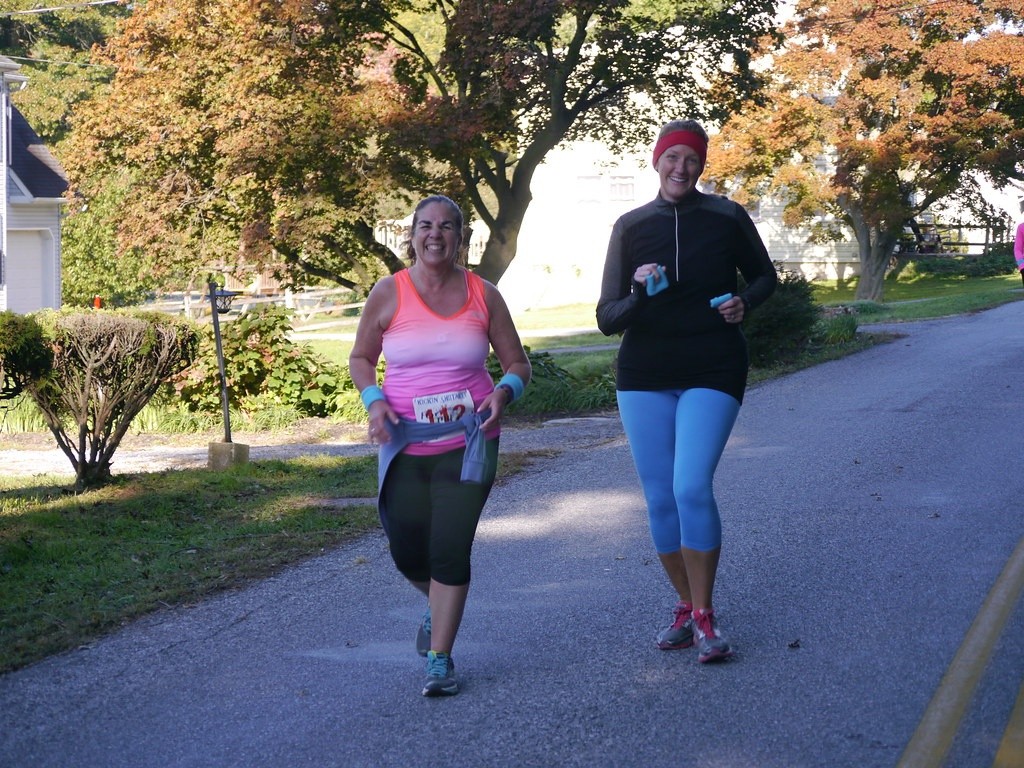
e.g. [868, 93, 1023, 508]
[203, 282, 239, 441]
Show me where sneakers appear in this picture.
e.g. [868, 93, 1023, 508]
[690, 608, 732, 663]
[421, 650, 458, 696]
[656, 600, 694, 650]
[414, 602, 432, 658]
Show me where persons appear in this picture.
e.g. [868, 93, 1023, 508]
[595, 121, 777, 664]
[349, 197, 532, 699]
[1014, 222, 1024, 290]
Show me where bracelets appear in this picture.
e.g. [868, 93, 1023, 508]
[645, 264, 669, 295]
[360, 385, 386, 411]
[710, 293, 732, 308]
[493, 371, 525, 405]
